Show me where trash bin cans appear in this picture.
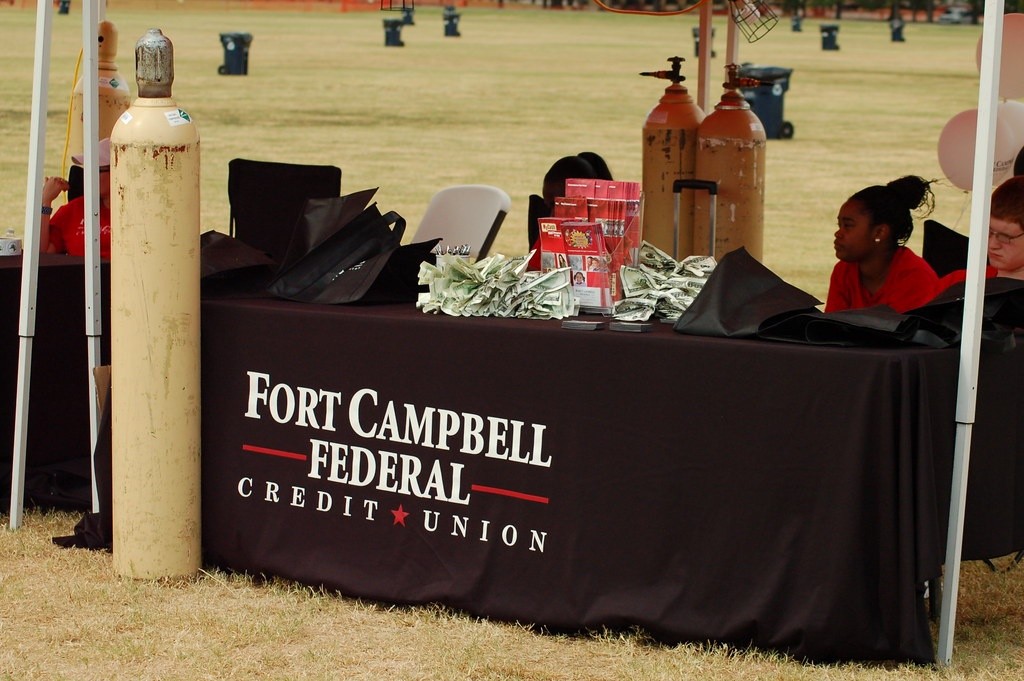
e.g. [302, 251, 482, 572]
[402, 7, 415, 24]
[58, 0, 70, 15]
[692, 26, 716, 58]
[737, 62, 793, 141]
[889, 18, 907, 42]
[791, 15, 804, 32]
[443, 4, 461, 37]
[820, 23, 840, 50]
[217, 32, 253, 76]
[382, 17, 406, 47]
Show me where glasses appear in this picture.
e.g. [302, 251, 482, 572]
[989, 226, 1024, 245]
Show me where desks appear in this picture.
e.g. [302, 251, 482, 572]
[1, 250, 1024, 669]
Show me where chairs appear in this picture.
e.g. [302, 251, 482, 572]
[223, 157, 342, 260]
[412, 181, 515, 266]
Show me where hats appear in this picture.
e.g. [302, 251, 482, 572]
[71, 137, 110, 167]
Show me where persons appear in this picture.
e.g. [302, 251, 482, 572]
[574, 271, 586, 288]
[586, 256, 593, 272]
[823, 173, 942, 322]
[547, 268, 552, 274]
[557, 253, 567, 268]
[525, 148, 622, 272]
[591, 257, 600, 272]
[903, 173, 1024, 332]
[39, 137, 115, 267]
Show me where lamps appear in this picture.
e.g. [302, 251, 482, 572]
[729, 0, 780, 43]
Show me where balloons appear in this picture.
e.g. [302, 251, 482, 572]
[988, 101, 1024, 199]
[935, 107, 1016, 193]
[973, 10, 1024, 103]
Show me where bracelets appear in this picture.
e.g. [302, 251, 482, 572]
[41, 204, 53, 217]
